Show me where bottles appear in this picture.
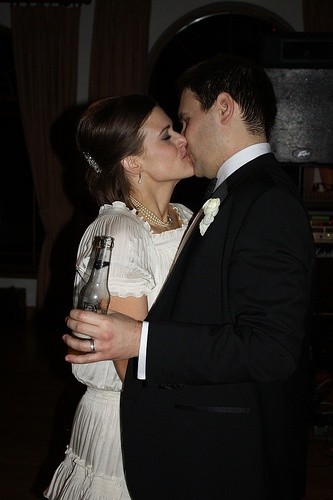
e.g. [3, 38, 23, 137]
[69, 234, 118, 356]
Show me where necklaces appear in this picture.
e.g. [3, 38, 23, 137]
[127, 195, 176, 228]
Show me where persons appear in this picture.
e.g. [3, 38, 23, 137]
[61, 52, 319, 499]
[40, 90, 197, 497]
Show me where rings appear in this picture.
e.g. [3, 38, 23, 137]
[88, 339, 98, 353]
